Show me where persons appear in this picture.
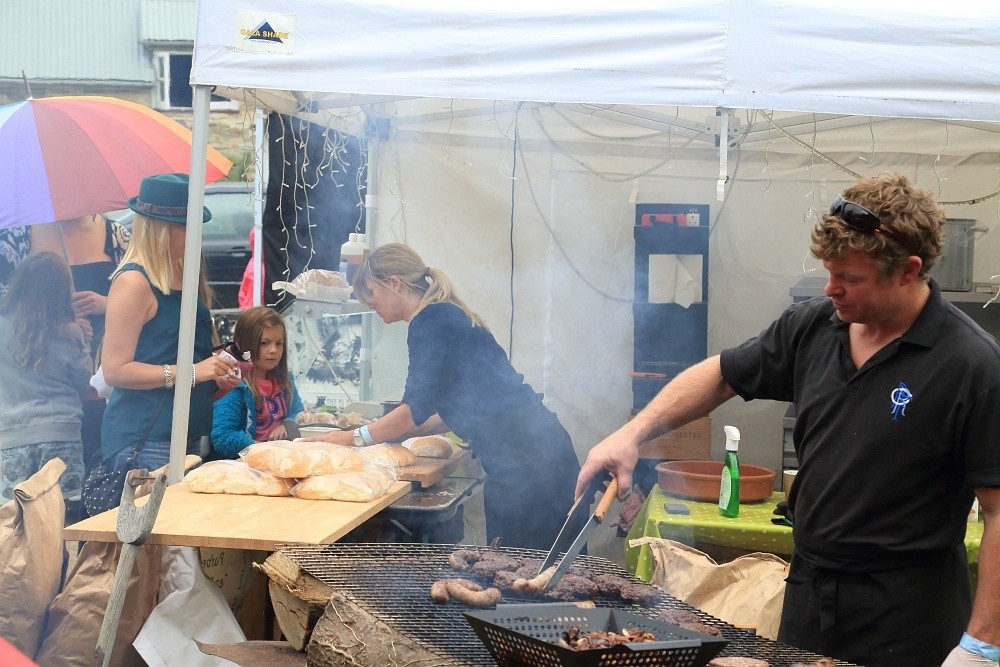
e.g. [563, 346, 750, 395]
[0, 212, 131, 476]
[575, 172, 1000, 667]
[210, 308, 305, 459]
[99, 174, 242, 487]
[297, 243, 587, 556]
[0, 263, 94, 526]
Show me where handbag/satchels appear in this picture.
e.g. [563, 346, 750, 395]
[83, 458, 141, 517]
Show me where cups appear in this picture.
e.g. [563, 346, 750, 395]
[783, 470, 798, 500]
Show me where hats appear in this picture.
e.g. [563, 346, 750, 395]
[128, 173, 212, 224]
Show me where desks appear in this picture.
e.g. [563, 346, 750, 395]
[621, 488, 981, 601]
[60, 477, 414, 553]
[381, 460, 494, 543]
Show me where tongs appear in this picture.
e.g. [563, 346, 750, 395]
[535, 469, 617, 593]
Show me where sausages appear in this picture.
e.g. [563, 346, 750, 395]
[430, 578, 502, 606]
[511, 567, 558, 598]
[448, 550, 481, 570]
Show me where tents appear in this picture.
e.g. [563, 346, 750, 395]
[162, 0, 1000, 482]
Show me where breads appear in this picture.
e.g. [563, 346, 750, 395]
[305, 270, 348, 288]
[182, 434, 452, 502]
[293, 410, 372, 429]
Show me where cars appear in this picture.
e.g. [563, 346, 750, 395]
[108, 182, 257, 310]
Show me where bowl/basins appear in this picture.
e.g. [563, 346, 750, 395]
[654, 460, 776, 501]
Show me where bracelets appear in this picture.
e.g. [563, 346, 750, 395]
[162, 364, 173, 389]
[360, 425, 375, 446]
[192, 364, 195, 389]
[959, 631, 1000, 662]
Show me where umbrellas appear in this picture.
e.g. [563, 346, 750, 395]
[0, 69, 235, 292]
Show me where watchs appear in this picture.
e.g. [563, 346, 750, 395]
[353, 429, 365, 447]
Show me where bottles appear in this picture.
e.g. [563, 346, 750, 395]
[338, 232, 371, 285]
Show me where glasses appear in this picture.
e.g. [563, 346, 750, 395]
[830, 193, 917, 255]
